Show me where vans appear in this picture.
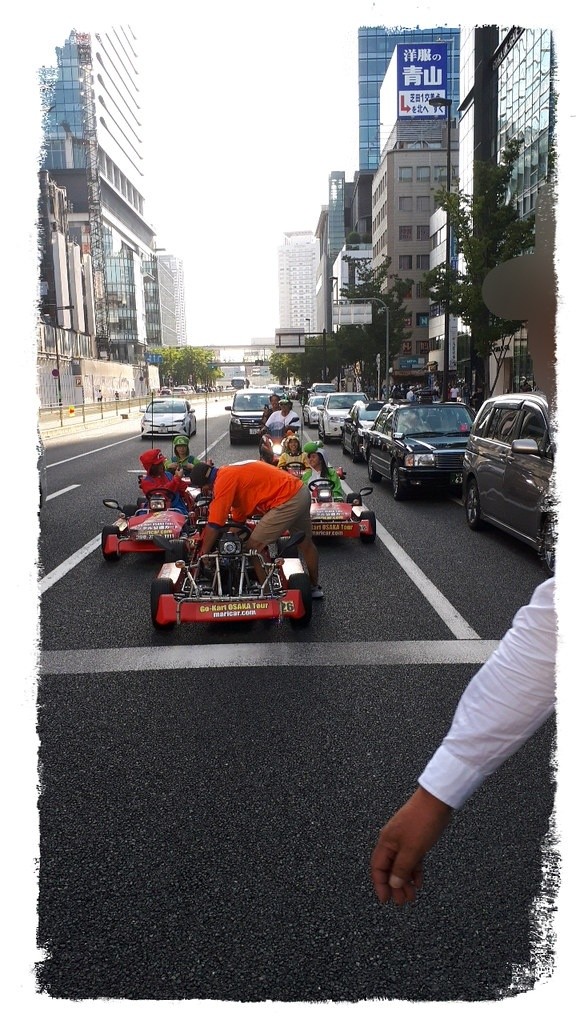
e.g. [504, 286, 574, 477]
[307, 382, 337, 401]
[317, 392, 370, 444]
[460, 389, 555, 579]
[224, 388, 275, 445]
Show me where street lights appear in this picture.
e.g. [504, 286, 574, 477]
[429, 97, 454, 403]
[53, 306, 74, 427]
[306, 318, 311, 338]
[329, 276, 340, 393]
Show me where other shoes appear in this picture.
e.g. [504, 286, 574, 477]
[309, 582, 323, 597]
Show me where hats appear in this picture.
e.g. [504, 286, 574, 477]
[189, 462, 213, 486]
[285, 436, 300, 447]
[152, 452, 165, 465]
[172, 436, 189, 445]
[410, 386, 414, 389]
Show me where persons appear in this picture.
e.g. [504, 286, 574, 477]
[300, 449, 344, 502]
[192, 461, 323, 597]
[97, 390, 102, 402]
[259, 394, 301, 438]
[131, 388, 135, 398]
[371, 575, 556, 903]
[382, 383, 417, 401]
[137, 449, 189, 526]
[115, 391, 119, 399]
[277, 435, 313, 471]
[164, 436, 200, 476]
[434, 381, 484, 412]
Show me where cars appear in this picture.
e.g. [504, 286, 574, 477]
[362, 397, 478, 501]
[159, 384, 300, 400]
[138, 398, 197, 441]
[339, 398, 412, 464]
[302, 395, 326, 428]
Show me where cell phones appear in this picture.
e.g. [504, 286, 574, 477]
[265, 404, 269, 410]
